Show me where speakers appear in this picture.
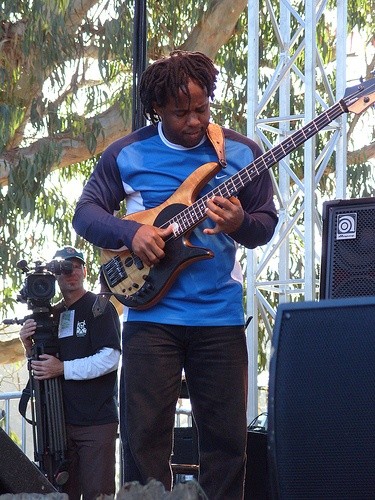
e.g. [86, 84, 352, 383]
[319, 197, 374, 298]
[0, 427, 60, 494]
[257, 298, 374, 499]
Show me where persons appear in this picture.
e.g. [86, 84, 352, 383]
[19, 246, 122, 500]
[72, 52, 279, 500]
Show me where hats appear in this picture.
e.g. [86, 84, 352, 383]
[53, 246, 85, 265]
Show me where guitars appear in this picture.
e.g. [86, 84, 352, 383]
[99, 69, 375, 310]
